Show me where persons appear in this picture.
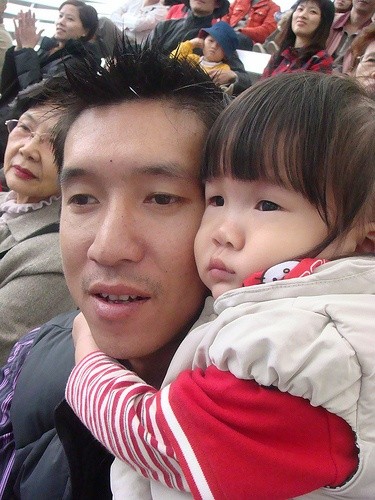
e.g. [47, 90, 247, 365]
[1, 0, 375, 192]
[62, 67, 375, 500]
[0, 41, 232, 500]
[0, 70, 85, 386]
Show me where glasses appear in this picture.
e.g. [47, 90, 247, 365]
[5, 119, 53, 145]
[356, 53, 375, 69]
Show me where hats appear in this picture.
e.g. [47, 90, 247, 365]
[183, 0, 230, 19]
[199, 21, 240, 62]
